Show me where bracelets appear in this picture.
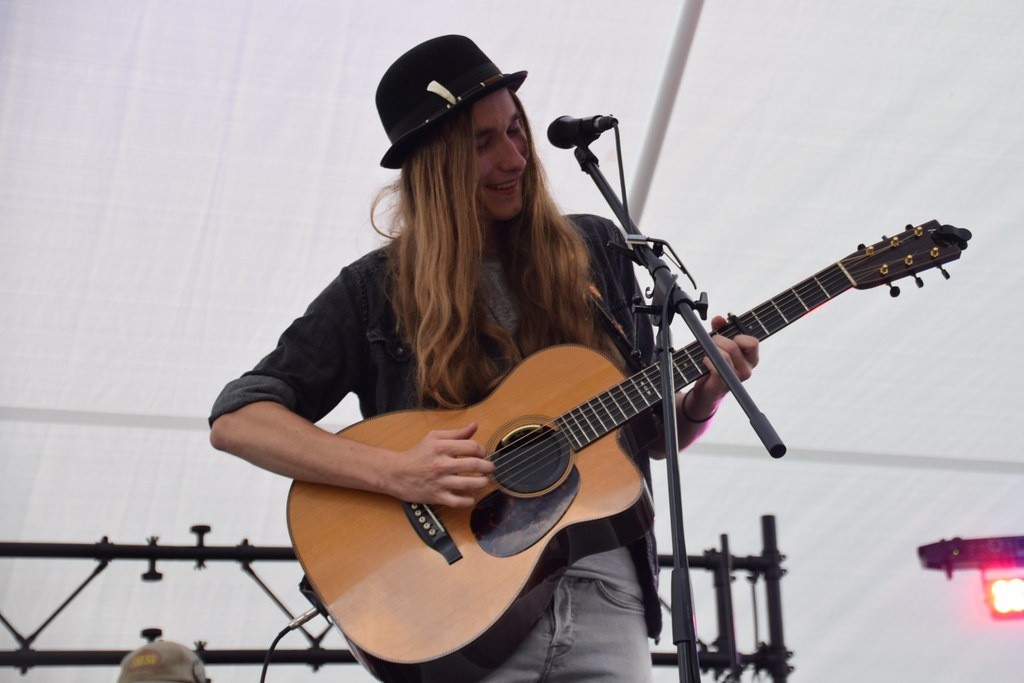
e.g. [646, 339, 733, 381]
[682, 388, 718, 424]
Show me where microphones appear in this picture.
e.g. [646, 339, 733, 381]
[547, 115, 619, 149]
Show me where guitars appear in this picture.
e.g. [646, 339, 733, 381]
[283, 214, 975, 683]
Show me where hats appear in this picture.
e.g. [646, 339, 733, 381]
[375, 35, 528, 169]
[116, 637, 208, 683]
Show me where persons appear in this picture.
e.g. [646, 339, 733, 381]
[207, 34, 760, 683]
